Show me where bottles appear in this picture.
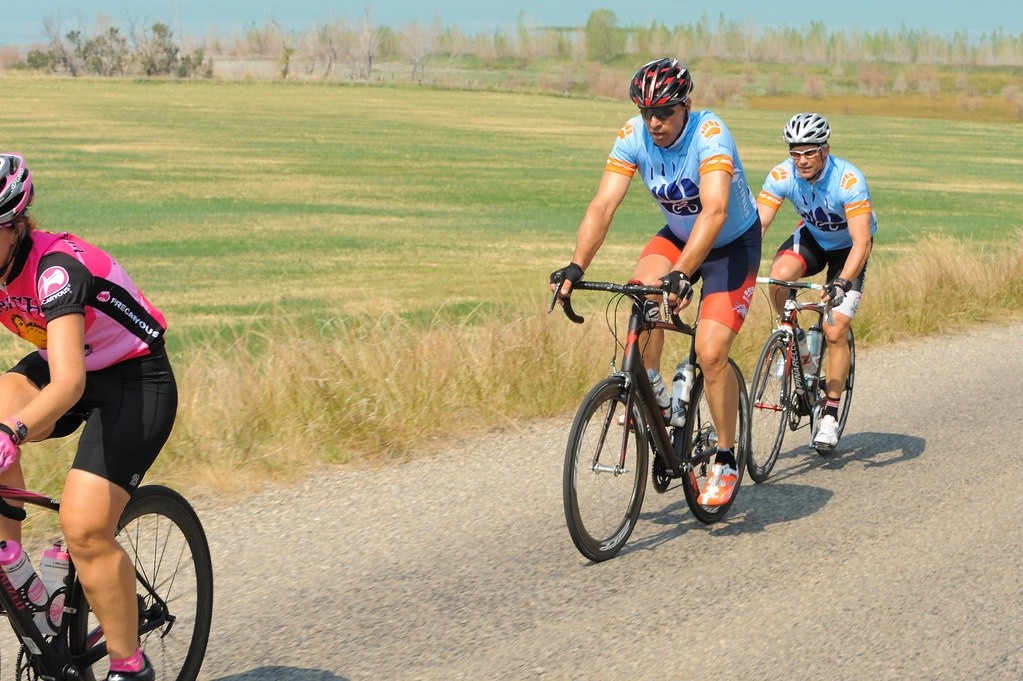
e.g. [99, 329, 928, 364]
[670, 362, 694, 427]
[806, 325, 823, 374]
[33, 544, 69, 636]
[647, 369, 672, 419]
[0, 540, 50, 614]
[796, 327, 811, 366]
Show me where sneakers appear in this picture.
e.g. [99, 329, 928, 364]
[695, 463, 739, 507]
[618, 404, 673, 425]
[813, 414, 839, 445]
[775, 354, 784, 377]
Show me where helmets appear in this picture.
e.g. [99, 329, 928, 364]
[629, 58, 693, 108]
[0, 152, 34, 224]
[782, 113, 830, 145]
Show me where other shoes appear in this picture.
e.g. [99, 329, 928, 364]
[106, 652, 156, 680]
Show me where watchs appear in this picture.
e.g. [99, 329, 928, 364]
[836, 278, 854, 292]
[8, 417, 27, 442]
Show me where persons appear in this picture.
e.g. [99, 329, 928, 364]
[757, 113, 877, 446]
[552, 55, 762, 506]
[0, 152, 178, 681]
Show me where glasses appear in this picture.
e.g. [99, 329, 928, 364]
[639, 103, 681, 120]
[789, 147, 821, 158]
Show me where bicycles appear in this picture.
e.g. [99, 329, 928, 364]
[747, 276, 855, 483]
[547, 270, 750, 562]
[0, 483, 214, 681]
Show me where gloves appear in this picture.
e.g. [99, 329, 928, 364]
[0, 423, 19, 474]
